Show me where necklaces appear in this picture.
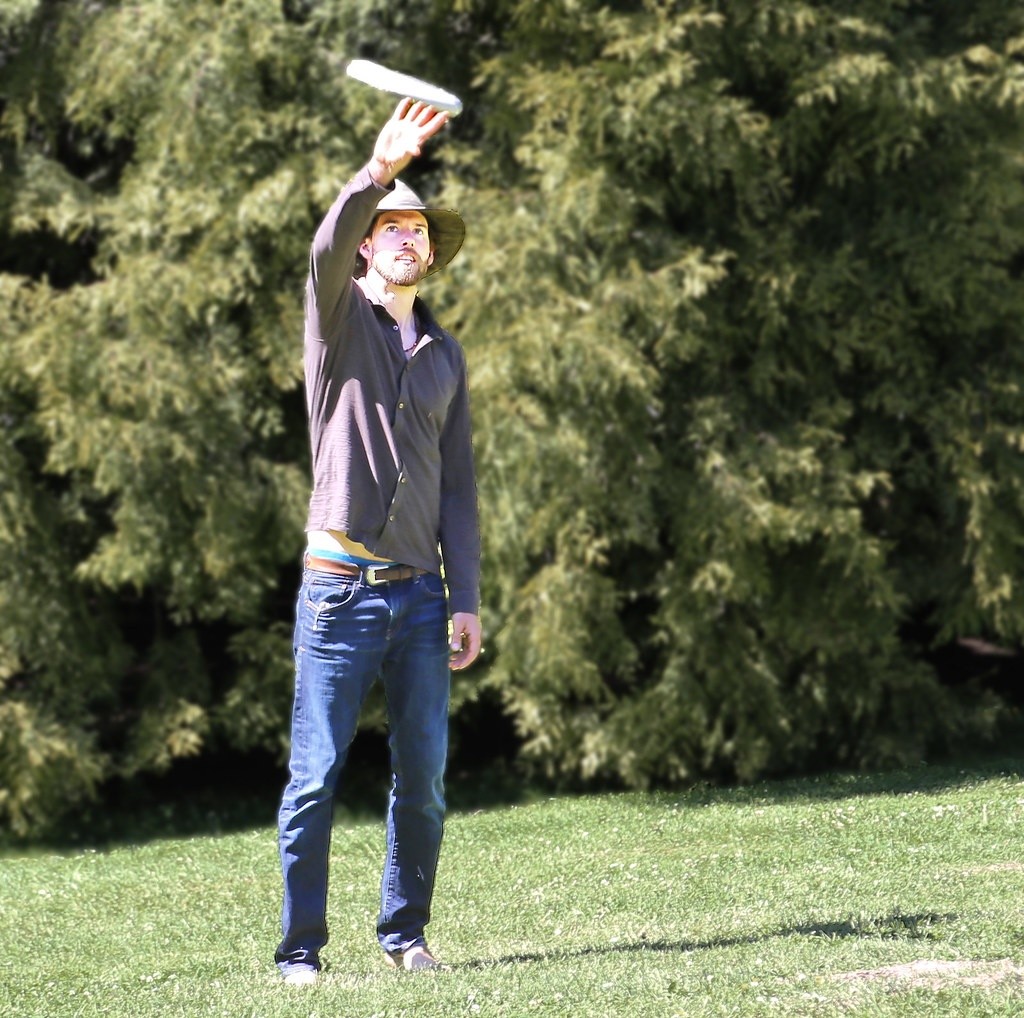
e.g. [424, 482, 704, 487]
[402, 309, 420, 352]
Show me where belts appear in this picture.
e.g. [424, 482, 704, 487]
[304, 552, 431, 588]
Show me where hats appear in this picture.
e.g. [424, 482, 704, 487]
[375, 177, 466, 280]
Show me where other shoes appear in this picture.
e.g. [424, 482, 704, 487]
[284, 969, 318, 988]
[384, 944, 453, 974]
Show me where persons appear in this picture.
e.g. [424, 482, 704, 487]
[274, 97, 481, 985]
